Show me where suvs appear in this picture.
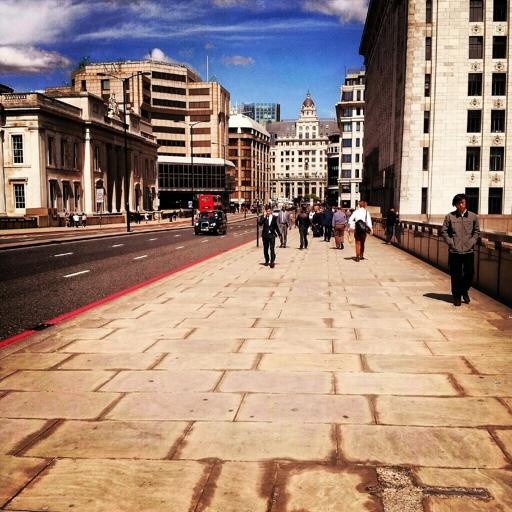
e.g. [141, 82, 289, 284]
[194, 210, 227, 236]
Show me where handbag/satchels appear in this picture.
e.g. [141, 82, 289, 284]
[355, 219, 371, 235]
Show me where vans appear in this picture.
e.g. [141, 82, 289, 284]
[272, 197, 294, 209]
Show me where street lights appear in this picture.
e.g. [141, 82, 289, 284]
[95, 71, 152, 233]
[211, 141, 238, 211]
[174, 119, 210, 227]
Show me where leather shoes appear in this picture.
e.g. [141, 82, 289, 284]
[326, 240, 330, 242]
[265, 261, 268, 266]
[462, 291, 470, 303]
[323, 240, 327, 241]
[453, 296, 461, 306]
[355, 256, 361, 262]
[341, 242, 344, 249]
[270, 262, 275, 268]
[337, 247, 340, 249]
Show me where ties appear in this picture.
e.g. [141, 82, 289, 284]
[283, 213, 286, 223]
[267, 215, 269, 227]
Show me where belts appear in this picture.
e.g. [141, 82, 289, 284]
[280, 223, 287, 224]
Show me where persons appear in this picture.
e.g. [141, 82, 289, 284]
[385, 207, 399, 244]
[258, 204, 283, 267]
[129, 210, 178, 225]
[348, 201, 373, 262]
[62, 210, 88, 228]
[218, 201, 354, 249]
[441, 194, 481, 306]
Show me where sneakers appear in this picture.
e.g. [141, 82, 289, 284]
[387, 241, 391, 244]
[383, 241, 387, 244]
